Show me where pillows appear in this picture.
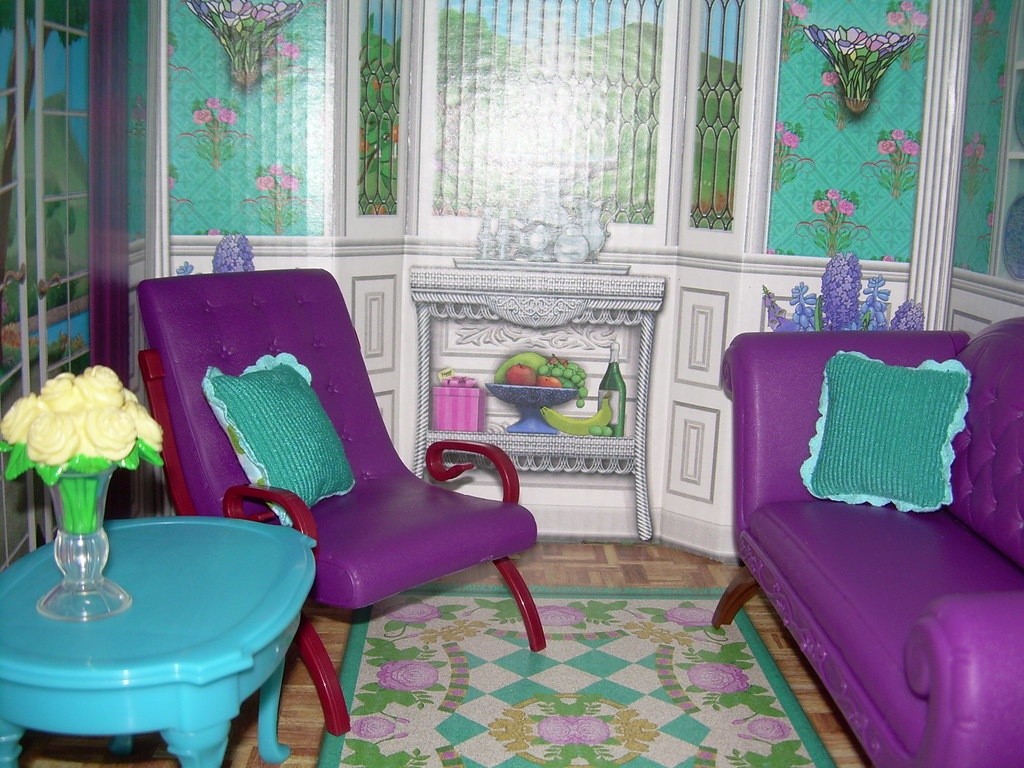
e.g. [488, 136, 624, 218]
[799, 348, 973, 515]
[201, 351, 357, 530]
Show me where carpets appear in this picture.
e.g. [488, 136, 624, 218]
[315, 581, 840, 768]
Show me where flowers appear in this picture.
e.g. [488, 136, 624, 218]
[0, 366, 166, 536]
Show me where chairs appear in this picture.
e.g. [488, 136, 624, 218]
[133, 266, 548, 738]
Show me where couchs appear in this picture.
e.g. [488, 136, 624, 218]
[711, 325, 1024, 768]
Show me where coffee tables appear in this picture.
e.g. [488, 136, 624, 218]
[1, 514, 320, 768]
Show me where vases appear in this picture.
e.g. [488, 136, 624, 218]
[35, 465, 134, 623]
